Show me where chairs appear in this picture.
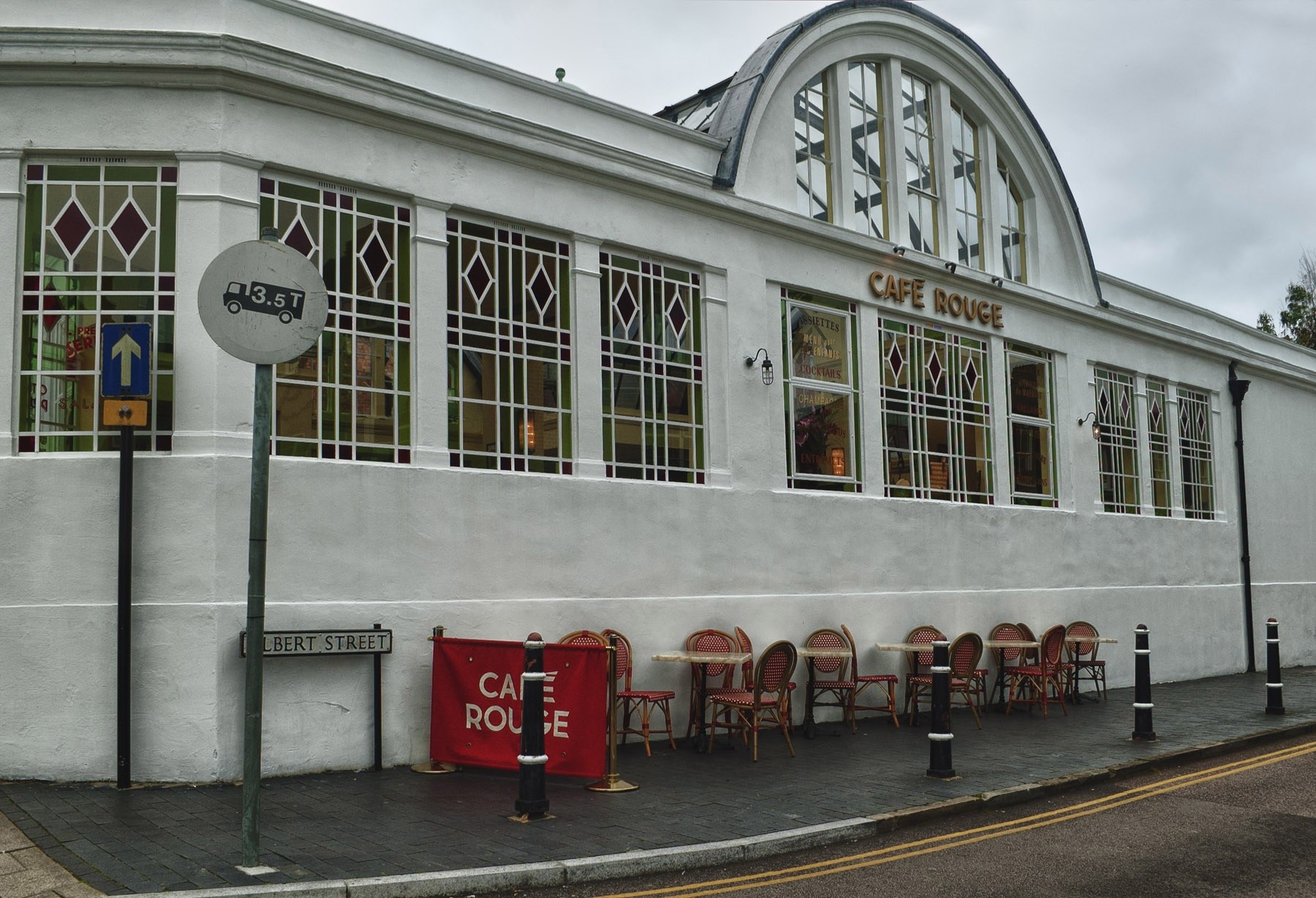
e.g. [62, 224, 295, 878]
[564, 618, 1120, 762]
